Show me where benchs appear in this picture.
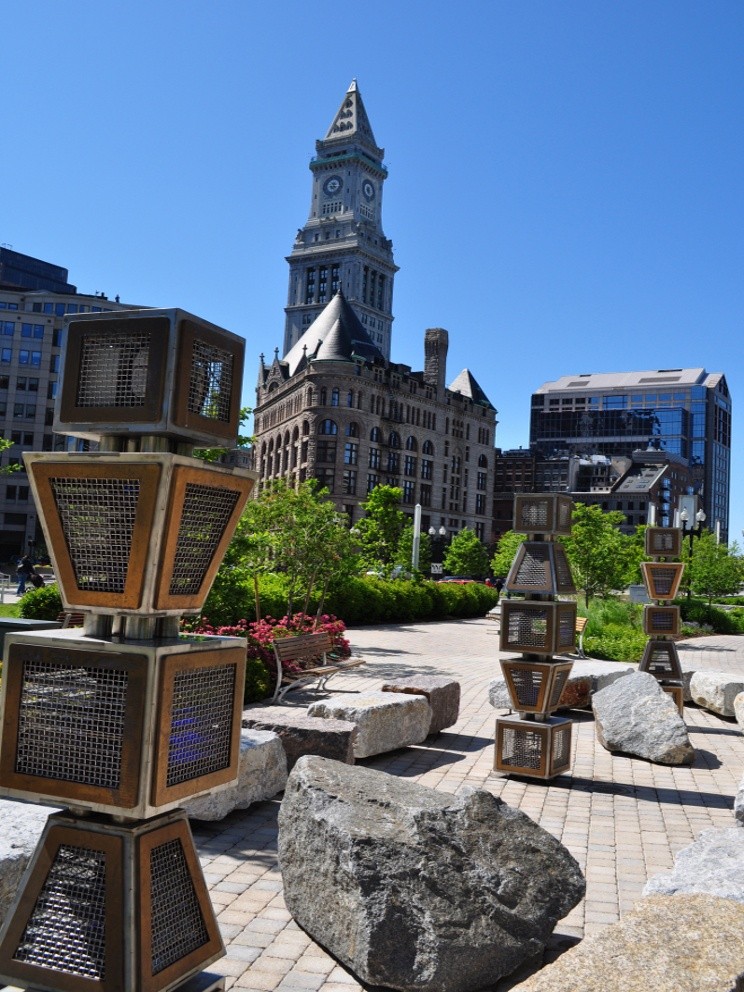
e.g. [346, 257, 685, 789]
[539, 616, 588, 659]
[271, 632, 366, 706]
[57, 611, 85, 630]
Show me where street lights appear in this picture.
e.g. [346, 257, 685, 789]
[427, 526, 447, 582]
[679, 504, 706, 601]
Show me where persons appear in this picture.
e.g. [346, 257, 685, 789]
[115, 295, 120, 302]
[16, 555, 34, 597]
[485, 578, 493, 587]
[493, 579, 502, 592]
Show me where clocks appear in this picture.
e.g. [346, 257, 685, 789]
[363, 179, 376, 202]
[323, 175, 343, 196]
